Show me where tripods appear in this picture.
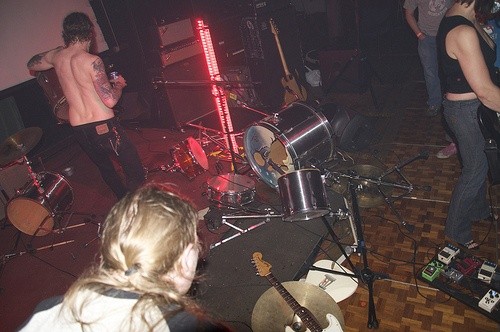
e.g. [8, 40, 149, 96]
[320, 149, 433, 231]
[20, 155, 96, 255]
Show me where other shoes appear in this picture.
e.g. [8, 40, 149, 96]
[483, 212, 499, 221]
[445, 229, 479, 250]
[436, 142, 458, 159]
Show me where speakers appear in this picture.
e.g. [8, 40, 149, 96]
[143, 37, 217, 129]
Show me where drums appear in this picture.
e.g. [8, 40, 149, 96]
[277, 169, 331, 221]
[36, 68, 71, 124]
[243, 102, 337, 193]
[169, 136, 210, 180]
[6, 171, 74, 237]
[205, 174, 256, 207]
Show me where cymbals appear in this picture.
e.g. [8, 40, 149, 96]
[0, 127, 43, 164]
[341, 164, 392, 208]
[252, 281, 345, 332]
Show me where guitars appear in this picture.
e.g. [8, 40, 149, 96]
[477, 67, 500, 143]
[251, 252, 343, 332]
[268, 18, 307, 104]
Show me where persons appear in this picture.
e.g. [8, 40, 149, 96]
[27, 12, 146, 201]
[436, 142, 458, 159]
[403, 0, 460, 113]
[436, 0, 500, 249]
[14, 179, 232, 332]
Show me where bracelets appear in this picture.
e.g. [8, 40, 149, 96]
[416, 31, 423, 37]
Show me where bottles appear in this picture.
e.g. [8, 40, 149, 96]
[108, 63, 119, 87]
